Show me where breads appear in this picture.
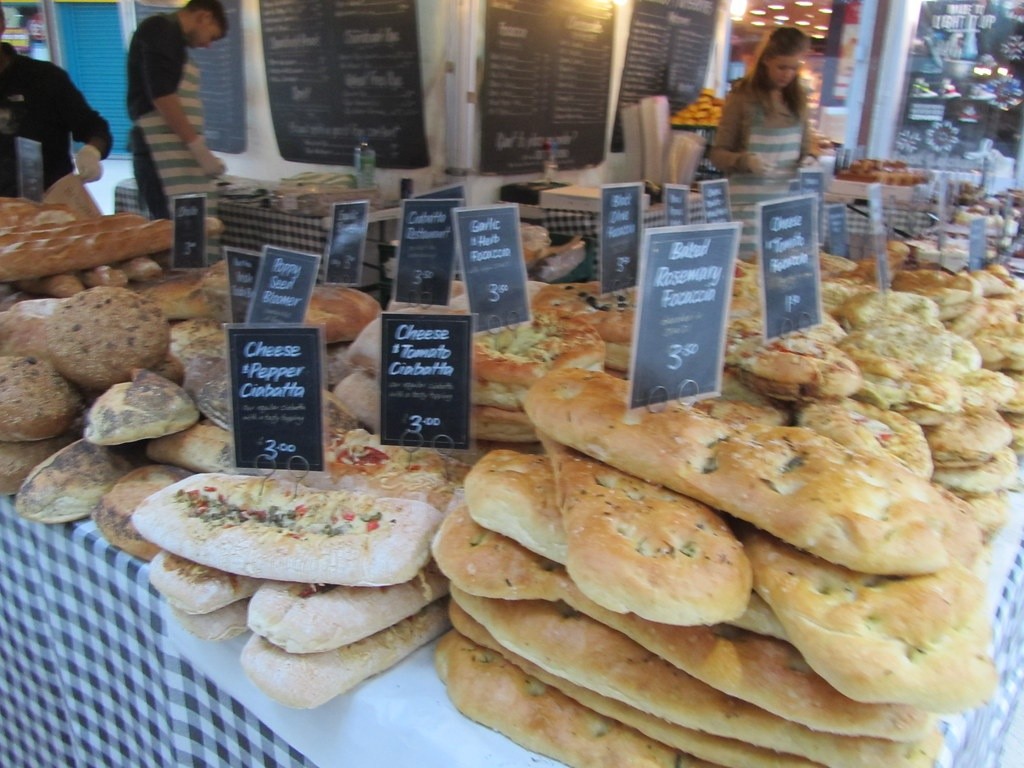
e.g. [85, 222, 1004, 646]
[669, 89, 726, 127]
[0, 160, 1024, 768]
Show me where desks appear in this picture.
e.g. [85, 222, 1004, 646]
[498, 193, 708, 286]
[839, 206, 928, 248]
[116, 173, 404, 309]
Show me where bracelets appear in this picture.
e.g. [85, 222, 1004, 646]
[806, 154, 817, 159]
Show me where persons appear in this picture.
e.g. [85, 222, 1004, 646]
[711, 27, 820, 265]
[126, 0, 230, 267]
[0, 4, 113, 204]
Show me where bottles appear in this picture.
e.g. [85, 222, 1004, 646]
[353, 142, 375, 189]
